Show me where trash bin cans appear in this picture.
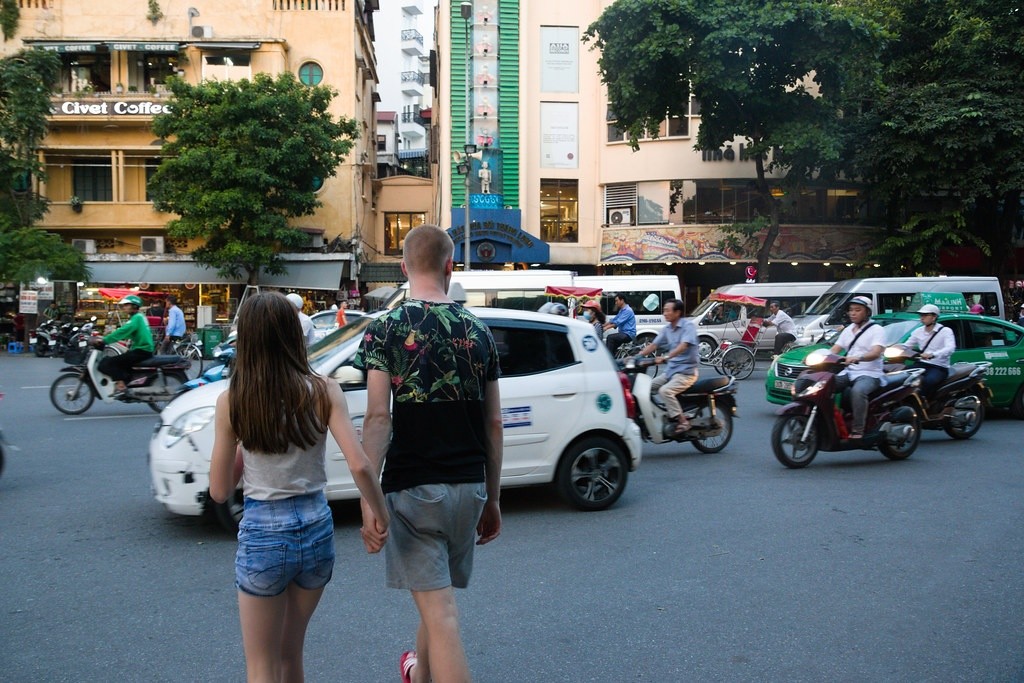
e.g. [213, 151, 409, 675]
[204, 329, 223, 360]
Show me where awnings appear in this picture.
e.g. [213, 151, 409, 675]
[27, 42, 100, 53]
[107, 42, 179, 51]
[48, 262, 344, 291]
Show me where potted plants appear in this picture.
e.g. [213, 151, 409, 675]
[70, 195, 83, 212]
[147, 85, 160, 98]
[116, 82, 123, 93]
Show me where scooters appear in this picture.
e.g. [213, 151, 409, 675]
[67, 316, 97, 351]
[27, 313, 57, 357]
[169, 336, 236, 402]
[48, 322, 75, 356]
[815, 323, 840, 344]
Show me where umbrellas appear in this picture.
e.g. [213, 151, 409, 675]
[364, 286, 398, 300]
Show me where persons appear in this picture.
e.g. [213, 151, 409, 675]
[478, 162, 492, 193]
[210, 291, 390, 683]
[93, 294, 155, 397]
[581, 300, 606, 340]
[326, 300, 337, 310]
[42, 302, 59, 322]
[762, 300, 797, 359]
[636, 299, 700, 434]
[11, 313, 28, 342]
[354, 225, 504, 683]
[829, 296, 887, 440]
[159, 297, 186, 355]
[716, 305, 737, 324]
[1001, 280, 1024, 323]
[336, 300, 347, 327]
[286, 293, 315, 349]
[601, 294, 636, 352]
[905, 304, 955, 409]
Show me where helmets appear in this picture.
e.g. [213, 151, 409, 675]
[118, 294, 142, 307]
[917, 304, 939, 316]
[849, 296, 872, 316]
[286, 293, 303, 309]
[581, 300, 601, 312]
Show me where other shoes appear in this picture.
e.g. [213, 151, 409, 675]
[108, 387, 128, 398]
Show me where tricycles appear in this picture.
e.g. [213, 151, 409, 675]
[698, 293, 808, 380]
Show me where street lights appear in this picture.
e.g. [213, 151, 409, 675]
[459, 1, 473, 270]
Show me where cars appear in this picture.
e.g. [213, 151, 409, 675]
[147, 305, 641, 536]
[765, 291, 1024, 421]
[304, 310, 368, 339]
[146, 307, 164, 326]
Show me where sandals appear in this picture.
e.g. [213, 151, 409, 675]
[675, 421, 691, 434]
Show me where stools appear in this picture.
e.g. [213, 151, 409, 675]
[0, 334, 35, 353]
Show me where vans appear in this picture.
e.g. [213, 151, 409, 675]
[398, 270, 682, 357]
[784, 276, 1006, 355]
[683, 282, 840, 361]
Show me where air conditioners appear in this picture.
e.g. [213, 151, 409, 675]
[299, 232, 323, 247]
[72, 238, 97, 255]
[141, 235, 165, 254]
[609, 207, 632, 227]
[191, 24, 212, 38]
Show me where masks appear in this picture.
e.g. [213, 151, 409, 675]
[583, 311, 593, 319]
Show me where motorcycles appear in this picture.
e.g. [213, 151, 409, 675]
[49, 315, 190, 415]
[613, 346, 740, 454]
[770, 348, 931, 470]
[881, 345, 994, 441]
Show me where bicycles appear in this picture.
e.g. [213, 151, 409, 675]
[152, 327, 204, 380]
[614, 341, 659, 379]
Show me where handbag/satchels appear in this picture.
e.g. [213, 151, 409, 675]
[832, 405, 849, 440]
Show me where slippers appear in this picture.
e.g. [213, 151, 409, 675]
[400, 650, 417, 683]
[848, 431, 863, 439]
